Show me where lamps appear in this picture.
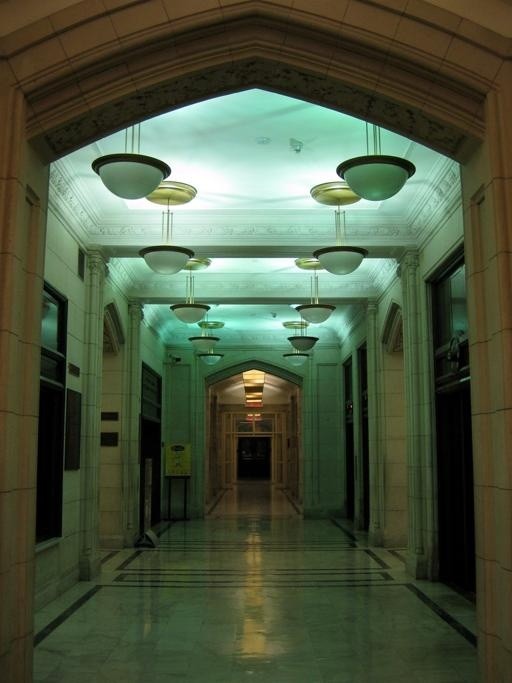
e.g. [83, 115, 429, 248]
[282, 123, 416, 367]
[90, 122, 223, 365]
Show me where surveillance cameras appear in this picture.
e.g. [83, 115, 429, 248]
[171, 354, 181, 362]
